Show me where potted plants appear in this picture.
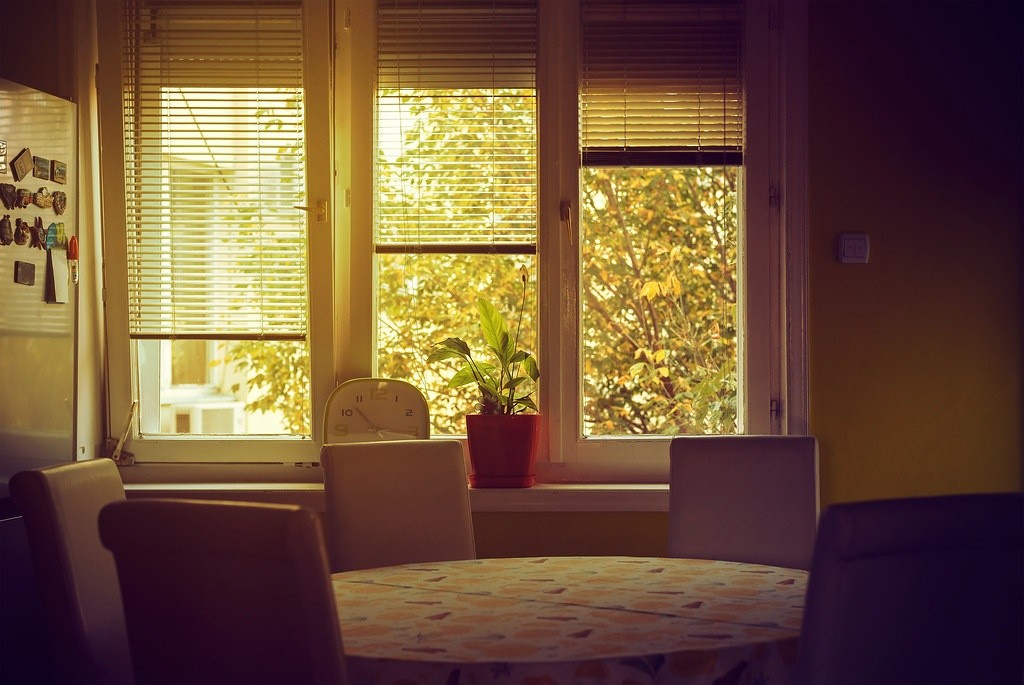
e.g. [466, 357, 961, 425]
[427, 263, 544, 490]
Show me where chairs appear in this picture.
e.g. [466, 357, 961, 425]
[314, 439, 477, 574]
[794, 491, 1024, 685]
[7, 456, 136, 685]
[669, 435, 820, 572]
[96, 499, 349, 685]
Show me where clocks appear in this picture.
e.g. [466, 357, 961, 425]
[320, 377, 431, 444]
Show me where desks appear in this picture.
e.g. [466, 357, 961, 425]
[329, 556, 809, 685]
